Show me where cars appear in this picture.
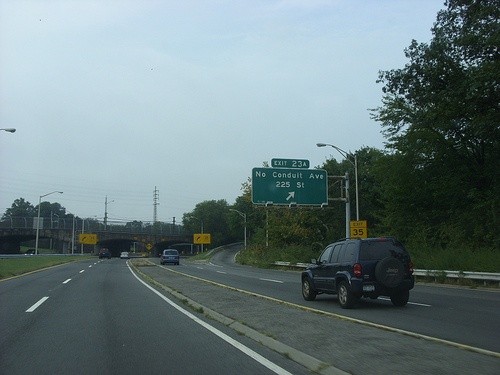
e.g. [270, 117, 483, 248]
[160, 248, 179, 265]
[121, 252, 130, 259]
[25, 249, 39, 256]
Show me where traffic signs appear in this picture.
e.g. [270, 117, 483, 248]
[249, 167, 329, 208]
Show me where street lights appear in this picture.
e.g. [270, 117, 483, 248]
[190, 216, 203, 253]
[229, 209, 247, 250]
[316, 142, 359, 220]
[104, 194, 115, 231]
[34, 191, 64, 254]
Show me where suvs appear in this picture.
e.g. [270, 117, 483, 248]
[301, 236, 415, 308]
[99, 249, 111, 259]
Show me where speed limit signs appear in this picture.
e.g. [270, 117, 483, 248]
[350, 220, 369, 240]
